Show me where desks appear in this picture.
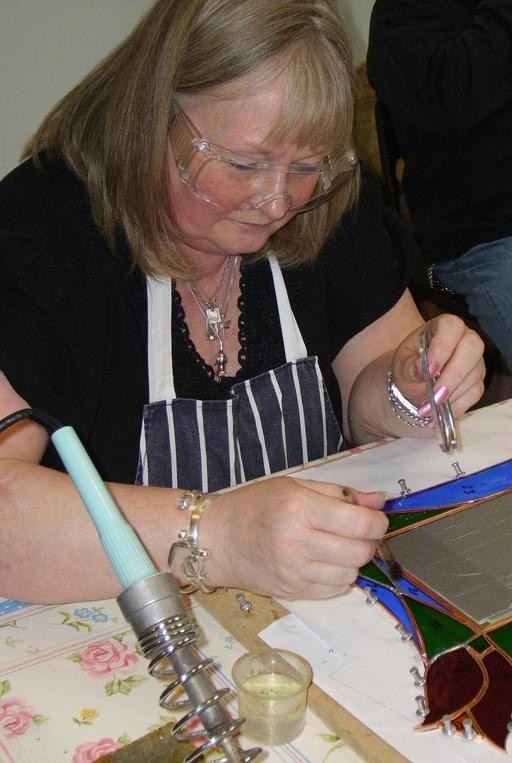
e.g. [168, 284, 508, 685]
[0, 398, 511, 763]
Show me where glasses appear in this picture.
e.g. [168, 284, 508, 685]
[166, 99, 357, 215]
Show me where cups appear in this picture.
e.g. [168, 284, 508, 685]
[230, 647, 313, 746]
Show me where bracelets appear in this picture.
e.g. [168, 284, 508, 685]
[163, 490, 217, 595]
[384, 363, 438, 430]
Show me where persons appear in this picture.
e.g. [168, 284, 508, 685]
[366, 0, 511, 383]
[2, 1, 490, 603]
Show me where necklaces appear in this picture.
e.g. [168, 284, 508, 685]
[174, 253, 245, 377]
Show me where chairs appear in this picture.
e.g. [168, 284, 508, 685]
[374, 100, 511, 391]
